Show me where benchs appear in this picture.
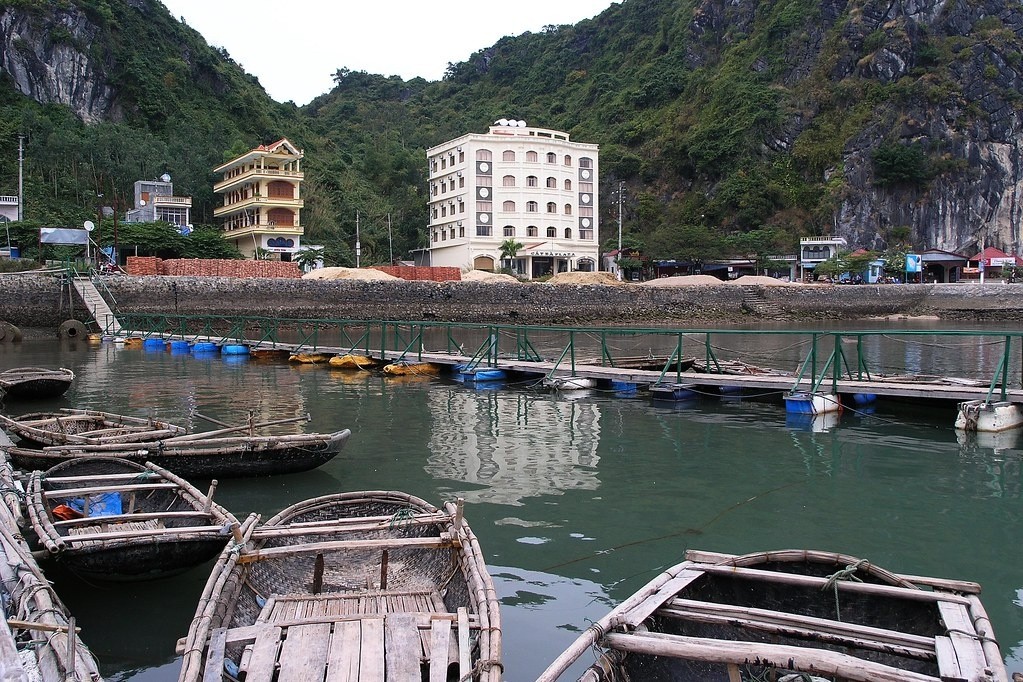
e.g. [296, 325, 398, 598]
[46, 473, 163, 483]
[601, 630, 944, 682]
[44, 482, 181, 497]
[249, 514, 454, 540]
[76, 426, 156, 438]
[54, 510, 212, 527]
[0, 370, 66, 377]
[236, 535, 464, 566]
[654, 595, 938, 661]
[20, 414, 105, 427]
[61, 525, 225, 542]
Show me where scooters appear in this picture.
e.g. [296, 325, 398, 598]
[852, 276, 866, 285]
[876, 273, 901, 284]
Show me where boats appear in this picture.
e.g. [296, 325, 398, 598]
[534, 547, 1009, 682]
[0, 407, 194, 447]
[0, 367, 76, 398]
[25, 456, 242, 595]
[174, 490, 505, 682]
[0, 410, 351, 481]
